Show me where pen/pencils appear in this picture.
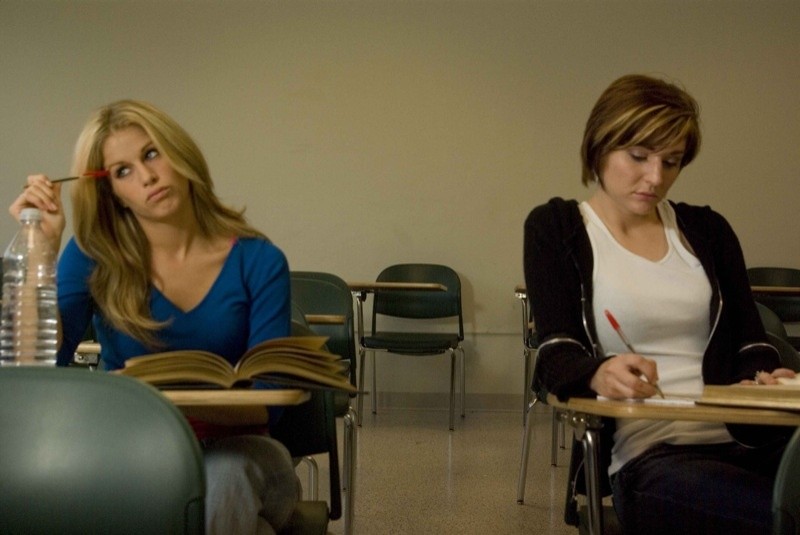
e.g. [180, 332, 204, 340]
[605, 310, 665, 398]
[24, 170, 111, 189]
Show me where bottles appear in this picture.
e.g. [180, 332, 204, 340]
[0, 208, 59, 366]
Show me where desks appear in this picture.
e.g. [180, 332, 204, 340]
[305, 314, 347, 325]
[161, 389, 309, 404]
[346, 282, 448, 292]
[546, 393, 800, 427]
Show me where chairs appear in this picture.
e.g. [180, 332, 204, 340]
[0, 263, 800, 535]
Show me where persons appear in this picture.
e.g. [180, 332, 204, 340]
[8, 102, 304, 534]
[524, 74, 800, 535]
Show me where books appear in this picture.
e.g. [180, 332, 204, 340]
[120, 334, 370, 396]
[596, 373, 800, 411]
